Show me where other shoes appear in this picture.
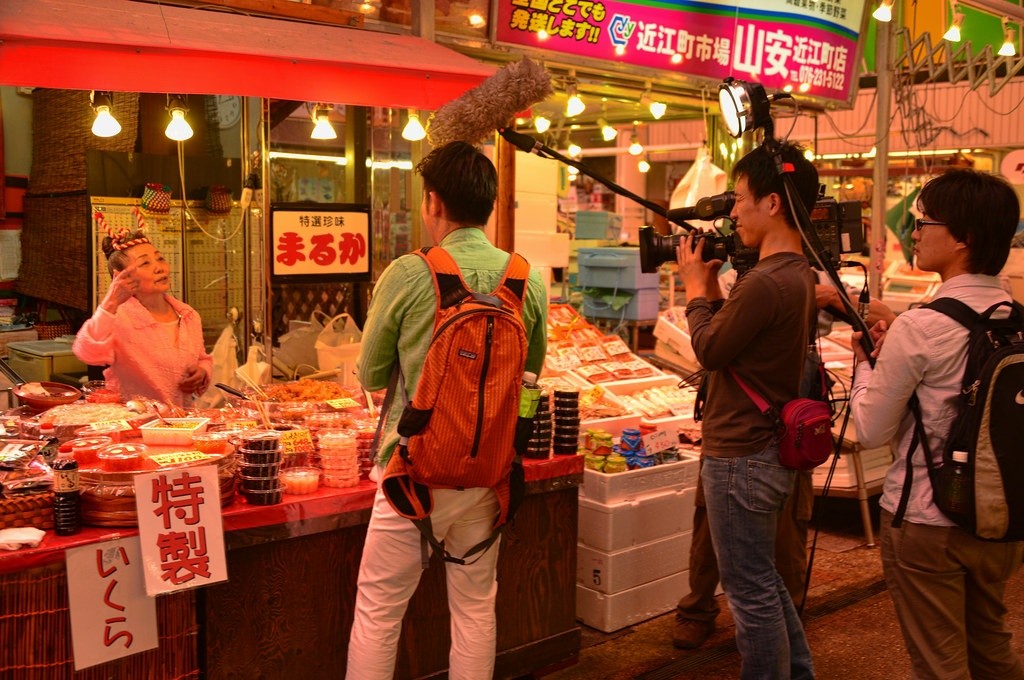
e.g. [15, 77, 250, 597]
[672, 614, 713, 648]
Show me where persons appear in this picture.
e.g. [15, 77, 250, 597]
[672, 138, 897, 680]
[346, 141, 548, 680]
[72, 233, 213, 409]
[849, 169, 1024, 680]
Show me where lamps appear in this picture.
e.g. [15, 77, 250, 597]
[165, 97, 193, 141]
[639, 89, 666, 119]
[597, 113, 618, 141]
[90, 91, 121, 137]
[403, 106, 427, 140]
[872, 0, 895, 22]
[718, 73, 771, 140]
[565, 138, 583, 158]
[565, 81, 586, 118]
[999, 18, 1017, 58]
[627, 127, 643, 157]
[311, 103, 337, 139]
[943, 0, 964, 43]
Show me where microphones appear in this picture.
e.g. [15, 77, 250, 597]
[664, 207, 695, 221]
[425, 57, 554, 150]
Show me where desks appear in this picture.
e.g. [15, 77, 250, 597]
[0, 452, 578, 680]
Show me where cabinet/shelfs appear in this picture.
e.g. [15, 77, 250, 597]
[6, 338, 90, 385]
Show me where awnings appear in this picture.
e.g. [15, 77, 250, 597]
[0, 0, 531, 119]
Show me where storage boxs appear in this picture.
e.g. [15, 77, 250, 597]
[577, 246, 659, 290]
[576, 452, 724, 633]
[651, 311, 705, 363]
[581, 286, 659, 320]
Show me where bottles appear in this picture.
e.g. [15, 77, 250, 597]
[52, 446, 81, 537]
[38, 423, 59, 467]
[521, 371, 538, 390]
[579, 422, 657, 473]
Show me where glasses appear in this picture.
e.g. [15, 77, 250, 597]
[914, 218, 947, 233]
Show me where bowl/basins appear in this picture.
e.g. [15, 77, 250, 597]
[527, 384, 581, 459]
[0, 379, 385, 506]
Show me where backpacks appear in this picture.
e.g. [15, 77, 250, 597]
[378, 247, 530, 533]
[910, 297, 1024, 543]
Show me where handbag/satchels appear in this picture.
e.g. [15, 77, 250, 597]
[776, 397, 833, 472]
[278, 308, 362, 389]
